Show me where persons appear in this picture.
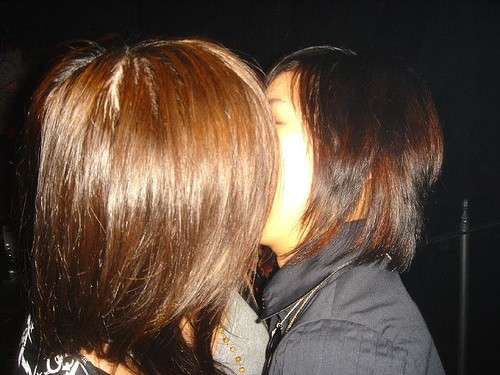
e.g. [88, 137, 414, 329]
[11, 39, 274, 375]
[258, 42, 449, 374]
